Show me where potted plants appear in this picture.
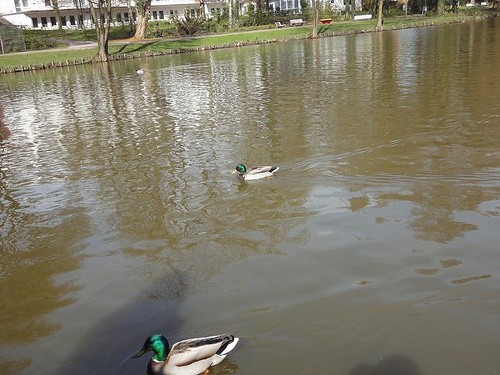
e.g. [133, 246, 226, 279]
[320, 18, 333, 25]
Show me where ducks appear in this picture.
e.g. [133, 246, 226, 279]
[231, 164, 280, 181]
[131, 331, 240, 375]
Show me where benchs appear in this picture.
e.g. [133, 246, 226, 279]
[352, 13, 373, 21]
[466, 3, 473, 7]
[289, 19, 306, 27]
[275, 21, 285, 29]
[481, 2, 488, 7]
